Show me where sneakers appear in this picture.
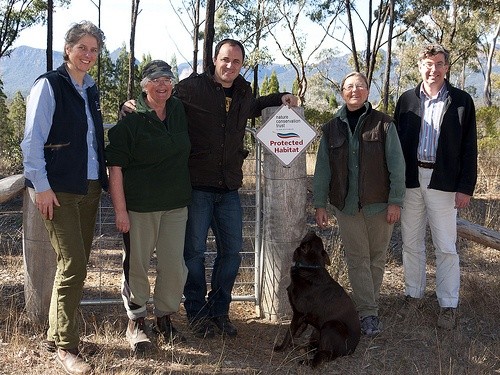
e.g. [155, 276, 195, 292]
[210, 306, 240, 338]
[436, 306, 458, 331]
[150, 314, 187, 346]
[124, 318, 156, 354]
[357, 315, 384, 338]
[46, 336, 97, 355]
[399, 295, 420, 316]
[188, 308, 216, 339]
[57, 347, 95, 375]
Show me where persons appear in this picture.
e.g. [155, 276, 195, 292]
[392, 46, 478, 331]
[18, 20, 110, 375]
[118, 39, 300, 341]
[106, 59, 189, 354]
[312, 71, 407, 336]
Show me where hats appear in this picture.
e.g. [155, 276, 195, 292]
[141, 59, 175, 82]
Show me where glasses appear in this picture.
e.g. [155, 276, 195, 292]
[342, 84, 369, 93]
[419, 61, 448, 69]
[145, 78, 174, 87]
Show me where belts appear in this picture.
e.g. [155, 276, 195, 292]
[417, 160, 438, 169]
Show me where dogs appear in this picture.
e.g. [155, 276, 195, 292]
[273, 227, 361, 369]
